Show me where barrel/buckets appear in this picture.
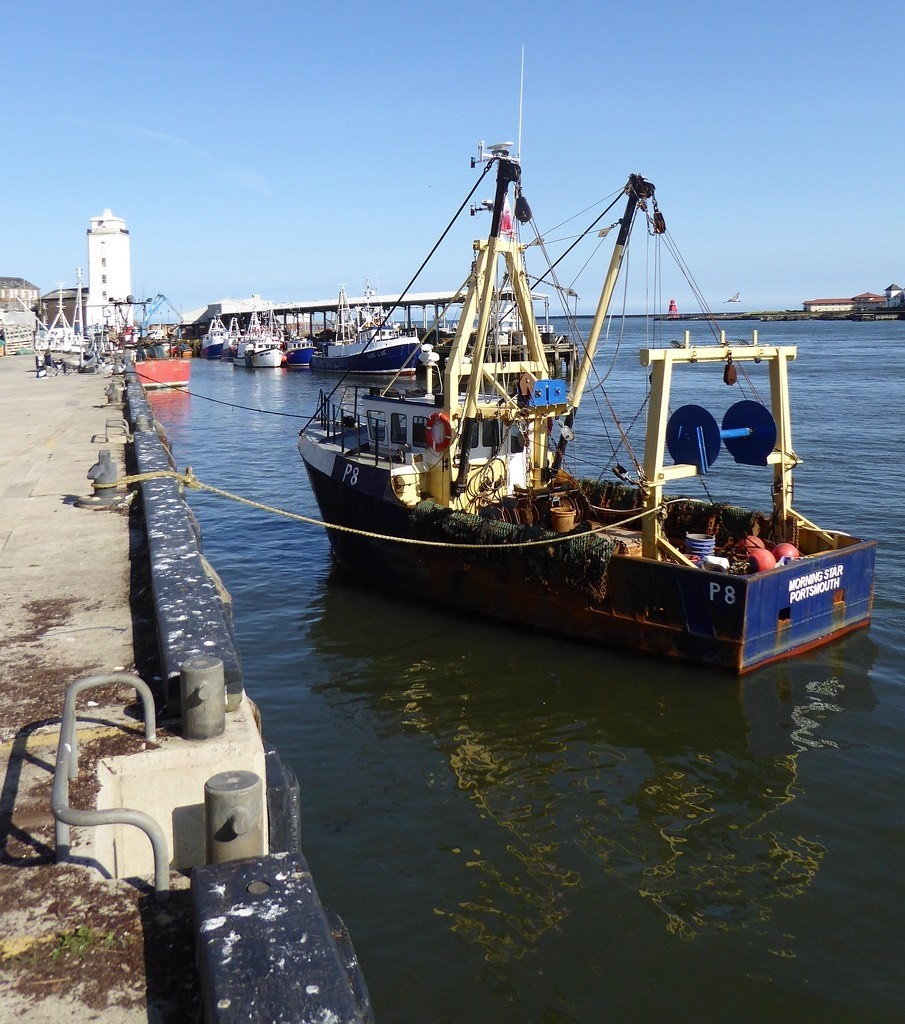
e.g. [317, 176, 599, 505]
[551, 507, 576, 532]
[685, 532, 716, 567]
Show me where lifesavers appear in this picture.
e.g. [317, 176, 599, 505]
[425, 412, 451, 452]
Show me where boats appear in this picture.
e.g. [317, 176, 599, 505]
[309, 276, 422, 375]
[199, 299, 317, 367]
[299, 44, 878, 674]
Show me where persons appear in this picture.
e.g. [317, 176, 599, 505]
[109, 326, 118, 347]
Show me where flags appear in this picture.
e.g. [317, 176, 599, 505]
[501, 196, 512, 237]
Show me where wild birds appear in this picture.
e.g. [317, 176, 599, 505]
[723, 292, 741, 303]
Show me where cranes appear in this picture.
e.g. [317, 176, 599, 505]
[140, 292, 184, 330]
